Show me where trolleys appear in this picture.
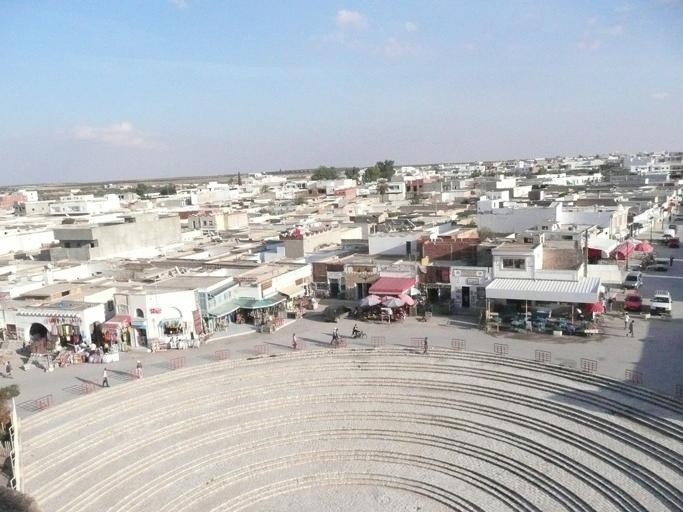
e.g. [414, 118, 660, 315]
[353, 330, 367, 339]
[336, 336, 348, 347]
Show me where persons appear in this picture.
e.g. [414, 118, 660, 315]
[291, 331, 298, 349]
[4, 362, 14, 378]
[421, 336, 429, 354]
[329, 326, 339, 344]
[135, 359, 144, 380]
[351, 323, 358, 336]
[623, 312, 630, 330]
[668, 254, 674, 266]
[625, 321, 636, 339]
[101, 367, 111, 387]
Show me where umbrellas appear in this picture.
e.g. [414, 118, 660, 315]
[580, 303, 603, 313]
[359, 287, 421, 310]
[633, 243, 653, 253]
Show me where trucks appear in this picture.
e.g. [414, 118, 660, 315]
[662, 228, 674, 243]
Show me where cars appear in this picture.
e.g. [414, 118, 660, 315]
[674, 214, 682, 220]
[667, 237, 679, 248]
[654, 258, 667, 271]
[622, 293, 642, 311]
[668, 225, 676, 229]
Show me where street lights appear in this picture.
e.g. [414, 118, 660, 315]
[624, 240, 630, 270]
[649, 216, 654, 243]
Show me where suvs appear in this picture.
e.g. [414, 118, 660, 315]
[648, 288, 672, 316]
[621, 271, 642, 289]
